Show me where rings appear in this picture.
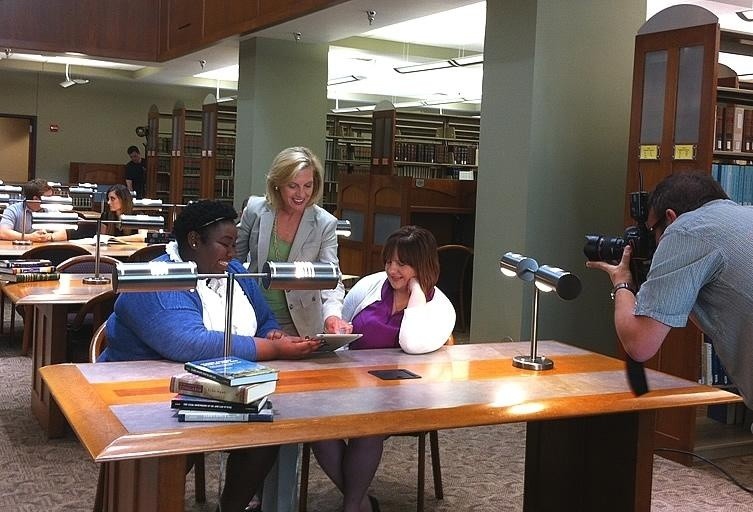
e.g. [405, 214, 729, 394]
[37, 238, 40, 243]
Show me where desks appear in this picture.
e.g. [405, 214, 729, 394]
[39, 339, 745, 510]
[0, 272, 113, 439]
[1, 240, 147, 355]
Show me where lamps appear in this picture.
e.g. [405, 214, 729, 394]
[391, 36, 483, 76]
[328, 75, 365, 88]
[58, 65, 89, 89]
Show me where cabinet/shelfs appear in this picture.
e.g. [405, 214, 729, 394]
[613, 6, 752, 466]
[67, 160, 127, 189]
[146, 93, 481, 237]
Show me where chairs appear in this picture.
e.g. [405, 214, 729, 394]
[0, 180, 475, 511]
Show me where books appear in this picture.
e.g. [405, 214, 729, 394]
[156, 119, 173, 196]
[183, 353, 280, 386]
[0, 273, 60, 282]
[215, 120, 236, 194]
[67, 233, 130, 248]
[398, 165, 477, 180]
[176, 409, 274, 423]
[1, 267, 57, 273]
[168, 371, 276, 404]
[144, 231, 175, 240]
[170, 393, 268, 413]
[324, 142, 372, 182]
[323, 182, 338, 215]
[183, 119, 202, 195]
[395, 142, 477, 165]
[0, 256, 52, 267]
[144, 237, 176, 245]
[699, 72, 752, 431]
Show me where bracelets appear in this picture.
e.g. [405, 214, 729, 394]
[608, 281, 637, 301]
[49, 232, 55, 242]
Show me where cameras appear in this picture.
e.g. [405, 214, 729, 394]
[583, 191, 651, 270]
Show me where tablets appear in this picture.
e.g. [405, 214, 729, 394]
[311, 333, 364, 353]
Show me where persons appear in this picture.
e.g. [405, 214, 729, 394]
[581, 163, 751, 423]
[123, 145, 149, 198]
[234, 143, 355, 512]
[98, 183, 141, 237]
[88, 197, 328, 512]
[0, 177, 67, 244]
[306, 223, 457, 512]
